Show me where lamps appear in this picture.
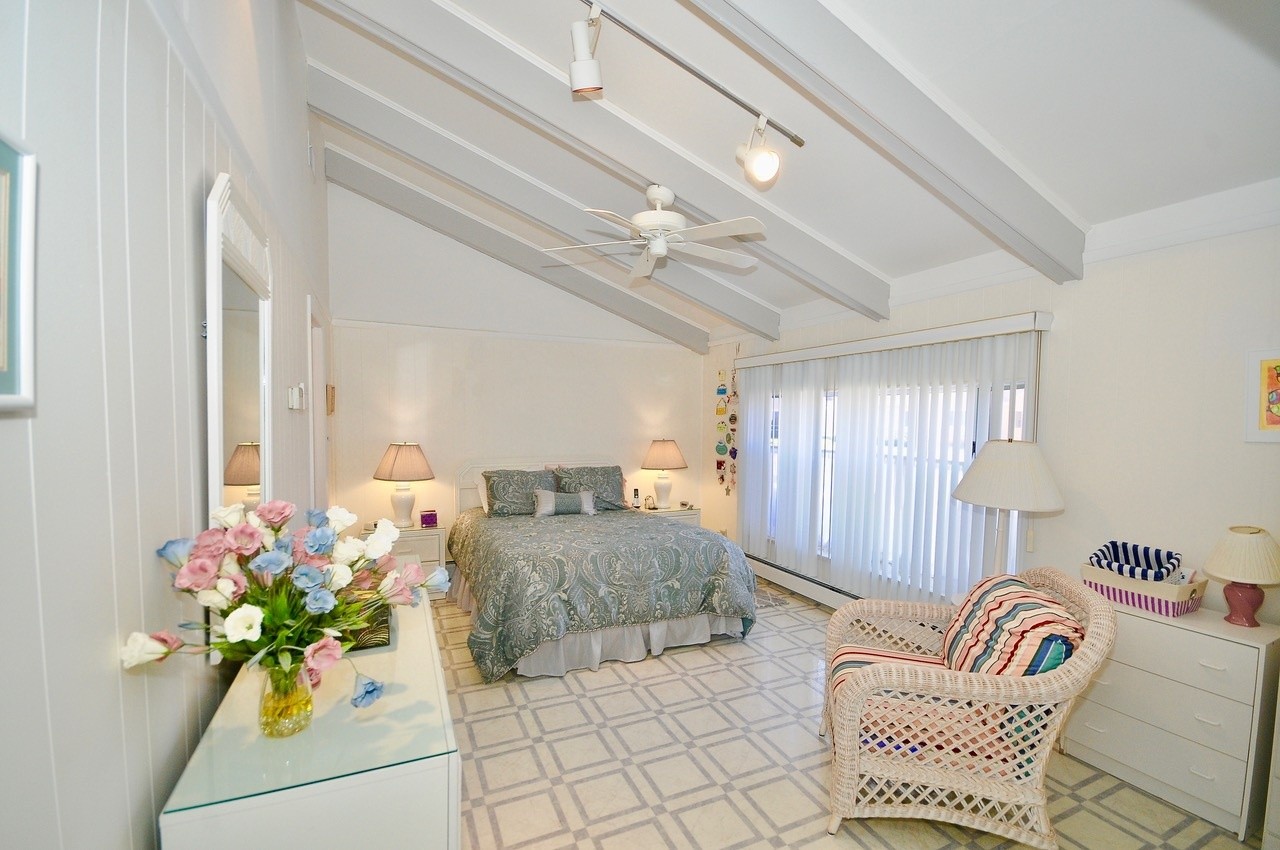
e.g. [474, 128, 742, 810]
[373, 442, 435, 526]
[568, 3, 604, 93]
[952, 438, 1065, 575]
[224, 442, 260, 515]
[1202, 526, 1280, 626]
[735, 116, 778, 181]
[640, 439, 687, 509]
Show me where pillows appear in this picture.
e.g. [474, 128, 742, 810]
[475, 465, 627, 517]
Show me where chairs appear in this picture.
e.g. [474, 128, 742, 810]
[820, 567, 1117, 850]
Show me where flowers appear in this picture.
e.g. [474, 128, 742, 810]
[119, 501, 452, 707]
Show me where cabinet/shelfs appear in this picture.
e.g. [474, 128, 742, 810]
[1056, 603, 1280, 840]
[157, 586, 463, 850]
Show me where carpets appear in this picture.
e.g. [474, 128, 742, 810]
[753, 588, 787, 608]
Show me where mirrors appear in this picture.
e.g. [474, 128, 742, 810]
[204, 173, 272, 663]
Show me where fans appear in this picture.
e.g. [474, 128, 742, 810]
[542, 185, 765, 278]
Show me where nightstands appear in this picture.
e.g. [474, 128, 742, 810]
[360, 527, 446, 600]
[638, 505, 701, 525]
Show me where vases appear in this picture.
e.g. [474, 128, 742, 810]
[259, 664, 315, 735]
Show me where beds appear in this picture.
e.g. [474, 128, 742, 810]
[448, 460, 756, 687]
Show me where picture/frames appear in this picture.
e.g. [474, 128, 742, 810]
[1244, 346, 1280, 444]
